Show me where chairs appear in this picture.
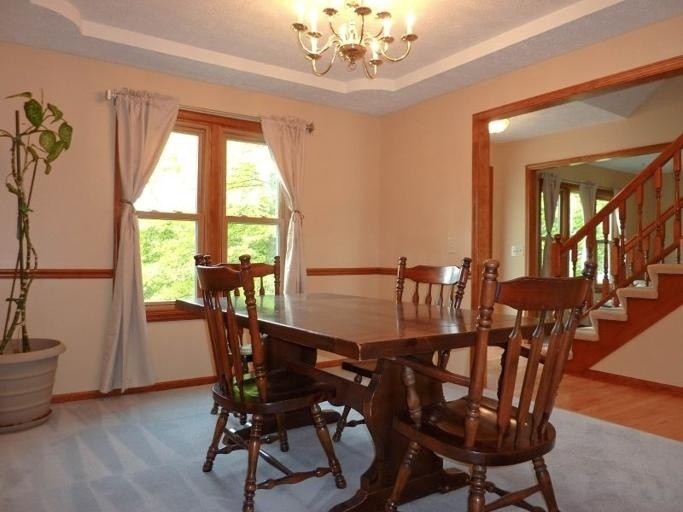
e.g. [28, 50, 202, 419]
[201, 253, 317, 417]
[382, 254, 596, 508]
[193, 253, 349, 508]
[331, 252, 475, 446]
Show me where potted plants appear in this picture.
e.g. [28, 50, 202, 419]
[0, 88, 76, 435]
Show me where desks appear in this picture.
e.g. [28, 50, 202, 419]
[173, 289, 563, 511]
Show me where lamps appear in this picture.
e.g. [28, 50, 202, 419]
[292, 5, 419, 82]
